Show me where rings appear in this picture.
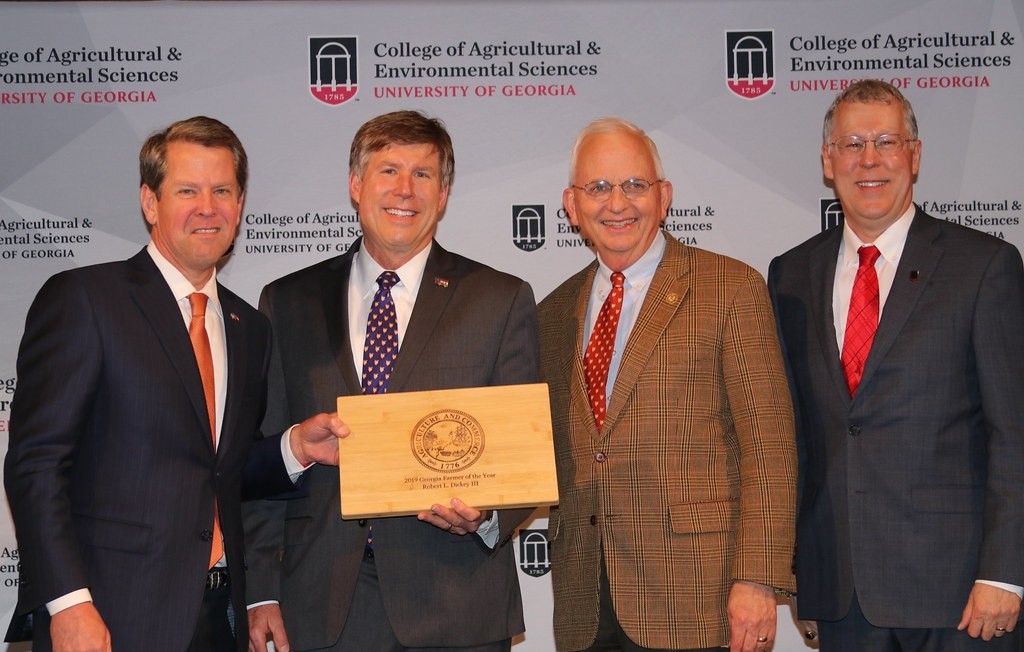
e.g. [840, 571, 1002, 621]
[996, 628, 1006, 631]
[757, 637, 767, 642]
[805, 631, 817, 640]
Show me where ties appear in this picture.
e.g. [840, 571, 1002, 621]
[361, 270, 399, 549]
[840, 245, 889, 400]
[181, 293, 224, 571]
[582, 271, 627, 436]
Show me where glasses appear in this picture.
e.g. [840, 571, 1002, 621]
[572, 178, 663, 203]
[826, 134, 920, 157]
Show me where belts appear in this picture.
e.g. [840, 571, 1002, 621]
[363, 548, 376, 565]
[204, 570, 231, 593]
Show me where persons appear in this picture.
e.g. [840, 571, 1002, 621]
[245, 111, 539, 652]
[6, 117, 346, 652]
[767, 79, 1024, 652]
[537, 117, 798, 652]
